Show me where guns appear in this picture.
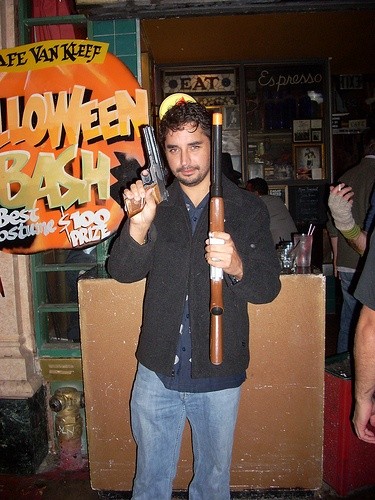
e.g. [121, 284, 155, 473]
[123, 125, 168, 218]
[209, 113, 225, 365]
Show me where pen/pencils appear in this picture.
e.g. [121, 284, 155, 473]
[307, 223, 316, 236]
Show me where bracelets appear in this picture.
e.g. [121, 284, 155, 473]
[341, 224, 360, 239]
[232, 276, 239, 283]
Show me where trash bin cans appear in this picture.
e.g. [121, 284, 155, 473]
[324, 348, 375, 499]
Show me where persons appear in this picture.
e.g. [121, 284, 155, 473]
[325, 139, 375, 445]
[246, 177, 300, 249]
[106, 100, 281, 500]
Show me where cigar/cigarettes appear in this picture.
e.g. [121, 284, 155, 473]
[338, 184, 341, 192]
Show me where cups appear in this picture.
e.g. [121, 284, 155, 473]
[293, 234, 313, 274]
